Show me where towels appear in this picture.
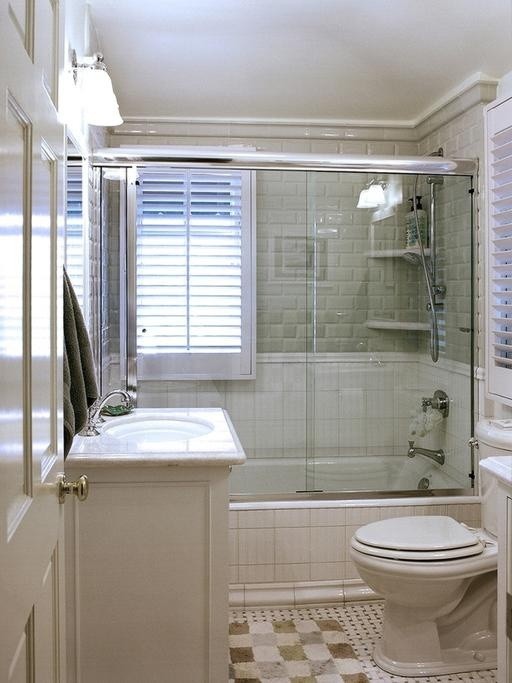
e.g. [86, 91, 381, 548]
[63, 267, 98, 460]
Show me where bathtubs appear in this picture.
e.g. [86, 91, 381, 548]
[227, 450, 470, 499]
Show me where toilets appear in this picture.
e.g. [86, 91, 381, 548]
[349, 415, 511, 677]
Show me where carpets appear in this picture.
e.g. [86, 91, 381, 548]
[228, 619, 370, 683]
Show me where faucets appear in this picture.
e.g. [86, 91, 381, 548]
[77, 387, 133, 438]
[404, 437, 446, 468]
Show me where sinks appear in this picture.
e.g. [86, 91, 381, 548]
[98, 410, 215, 448]
